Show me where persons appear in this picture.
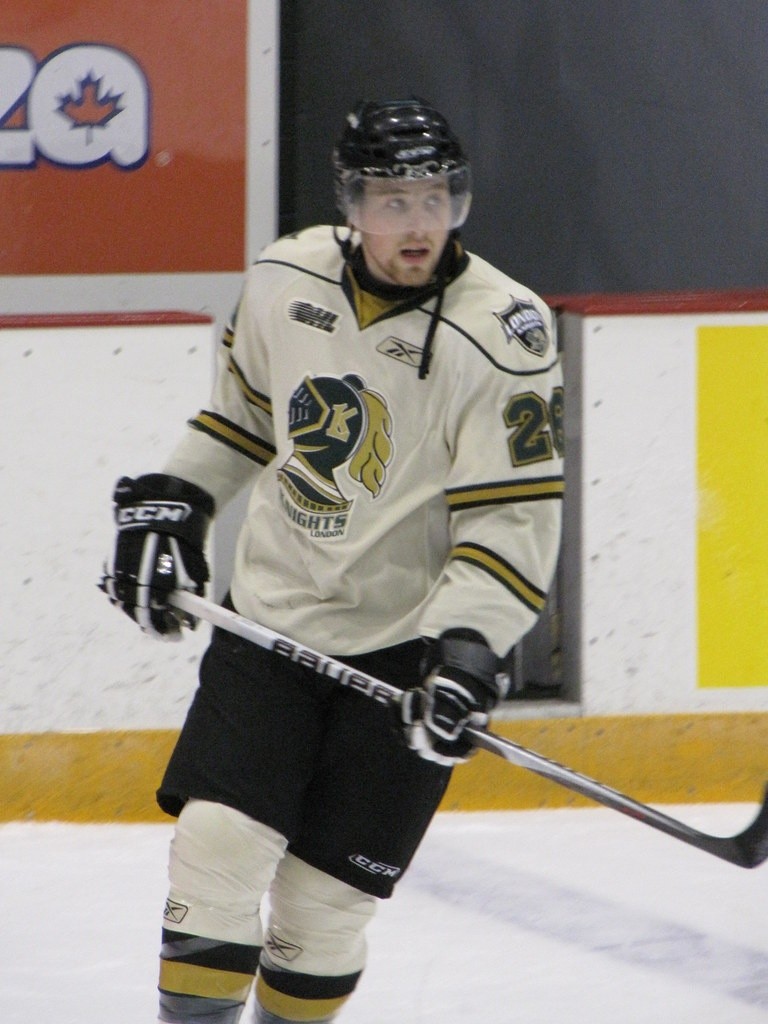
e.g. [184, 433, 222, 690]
[105, 89, 565, 1023]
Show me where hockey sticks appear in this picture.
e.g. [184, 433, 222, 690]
[104, 559, 768, 868]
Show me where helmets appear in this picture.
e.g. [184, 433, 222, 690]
[331, 96, 474, 215]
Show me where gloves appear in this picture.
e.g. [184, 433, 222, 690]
[399, 626, 511, 769]
[103, 472, 216, 643]
